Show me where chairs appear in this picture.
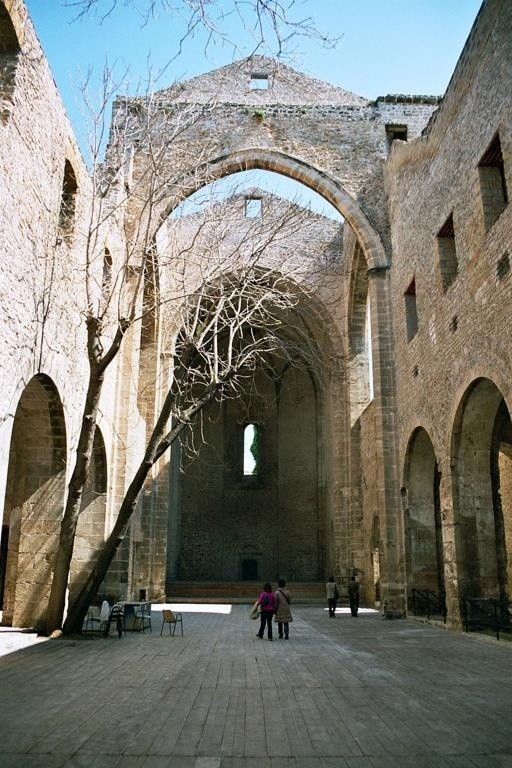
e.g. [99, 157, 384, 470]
[84, 601, 127, 639]
[160, 609, 183, 638]
[132, 606, 152, 633]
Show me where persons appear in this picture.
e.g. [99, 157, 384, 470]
[324, 575, 340, 618]
[252, 583, 276, 641]
[274, 580, 294, 639]
[347, 576, 360, 617]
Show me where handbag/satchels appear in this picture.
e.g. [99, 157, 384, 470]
[261, 592, 269, 607]
[248, 601, 262, 619]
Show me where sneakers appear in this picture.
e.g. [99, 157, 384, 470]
[352, 614, 358, 617]
[329, 613, 336, 618]
[255, 634, 273, 641]
[279, 632, 289, 640]
